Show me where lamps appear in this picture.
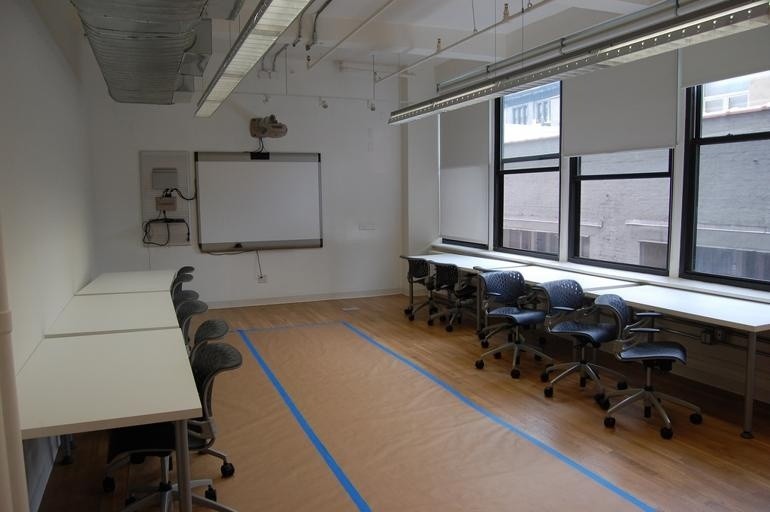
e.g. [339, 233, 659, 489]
[196, 0, 316, 119]
[387, 2, 770, 126]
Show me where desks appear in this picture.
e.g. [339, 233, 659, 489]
[490, 264, 641, 294]
[583, 285, 770, 439]
[406, 252, 528, 270]
[17, 269, 203, 512]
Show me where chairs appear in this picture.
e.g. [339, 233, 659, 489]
[427, 259, 487, 332]
[591, 292, 688, 440]
[404, 257, 454, 324]
[102, 267, 242, 512]
[528, 278, 631, 410]
[472, 270, 555, 378]
